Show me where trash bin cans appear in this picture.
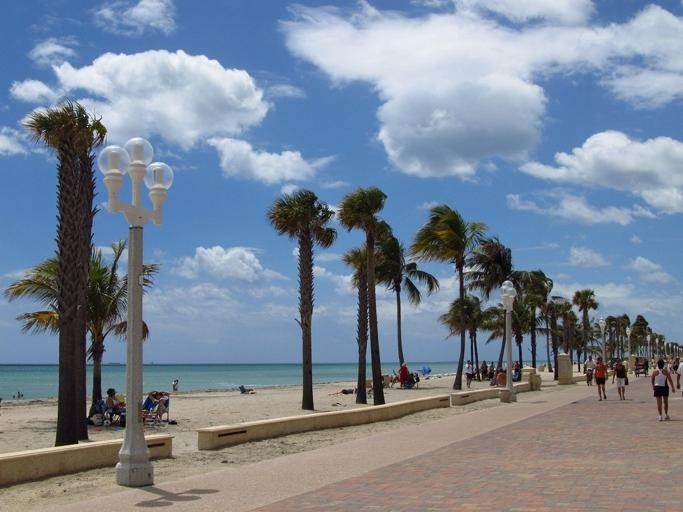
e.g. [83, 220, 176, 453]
[539, 365, 544, 372]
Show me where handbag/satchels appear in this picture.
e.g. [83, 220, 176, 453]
[92, 414, 103, 426]
[625, 378, 628, 385]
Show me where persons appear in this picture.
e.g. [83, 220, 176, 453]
[172, 380, 177, 391]
[141, 391, 169, 412]
[594, 356, 609, 401]
[479, 359, 520, 388]
[463, 359, 473, 390]
[148, 392, 170, 425]
[326, 386, 357, 395]
[611, 358, 627, 400]
[651, 358, 675, 421]
[583, 355, 596, 387]
[389, 369, 398, 389]
[238, 384, 256, 394]
[398, 361, 409, 389]
[105, 388, 126, 416]
[611, 353, 682, 378]
[675, 361, 682, 398]
[408, 371, 420, 389]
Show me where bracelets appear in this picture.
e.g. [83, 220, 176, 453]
[676, 383, 679, 386]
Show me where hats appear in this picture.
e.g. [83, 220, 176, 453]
[108, 391, 118, 395]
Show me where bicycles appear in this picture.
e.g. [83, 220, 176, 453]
[584, 367, 595, 385]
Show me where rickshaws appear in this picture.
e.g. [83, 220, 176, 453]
[635, 356, 649, 377]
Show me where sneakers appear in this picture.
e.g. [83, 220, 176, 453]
[658, 415, 663, 421]
[664, 415, 670, 419]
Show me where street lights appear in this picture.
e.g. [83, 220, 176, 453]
[663, 340, 682, 358]
[499, 280, 516, 389]
[599, 316, 607, 362]
[626, 326, 631, 369]
[656, 337, 659, 356]
[96, 137, 174, 461]
[645, 334, 651, 361]
